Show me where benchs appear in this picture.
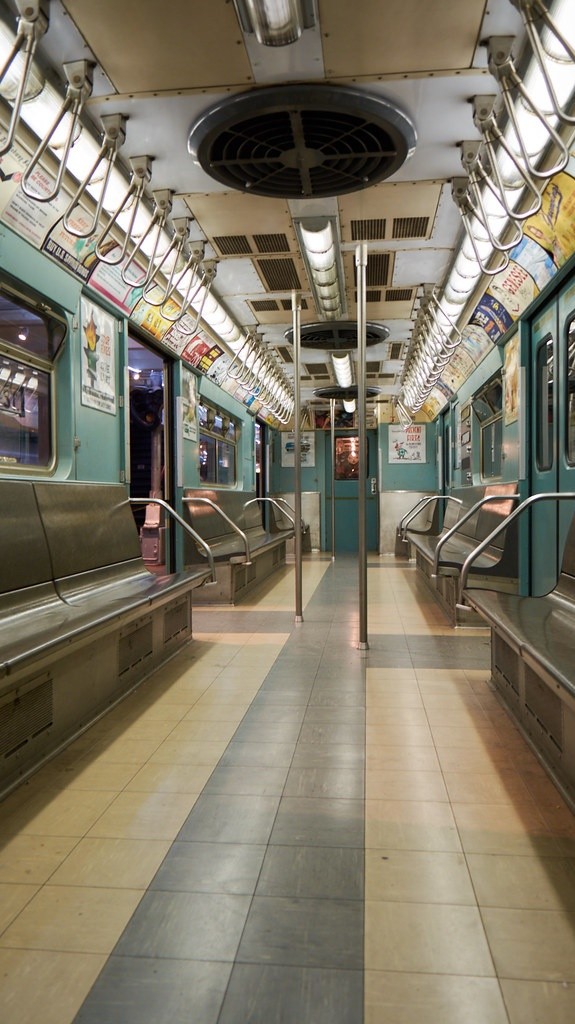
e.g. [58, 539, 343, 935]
[458, 490, 575, 818]
[268, 494, 311, 540]
[0, 476, 221, 804]
[186, 487, 297, 605]
[394, 491, 439, 562]
[401, 481, 518, 631]
